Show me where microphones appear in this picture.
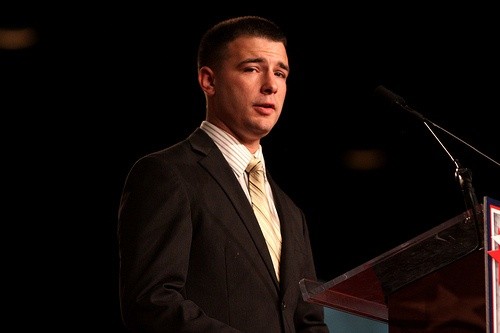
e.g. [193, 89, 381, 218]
[377, 85, 500, 210]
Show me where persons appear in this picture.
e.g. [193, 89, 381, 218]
[117, 17, 330, 333]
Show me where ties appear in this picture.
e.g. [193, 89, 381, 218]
[245, 157, 282, 282]
